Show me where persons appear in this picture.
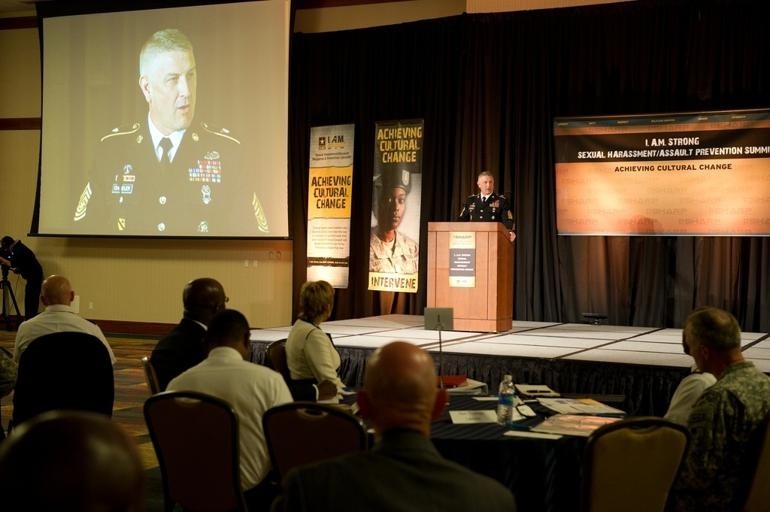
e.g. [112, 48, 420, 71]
[147, 277, 516, 511]
[11, 275, 117, 366]
[662, 305, 718, 430]
[665, 306, 770, 511]
[456, 171, 517, 243]
[71, 28, 274, 235]
[0, 236, 44, 322]
[352, 171, 421, 275]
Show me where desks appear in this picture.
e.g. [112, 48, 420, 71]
[341, 387, 628, 512]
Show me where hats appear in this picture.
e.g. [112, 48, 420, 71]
[372, 163, 412, 192]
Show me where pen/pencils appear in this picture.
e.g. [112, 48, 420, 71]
[513, 424, 531, 428]
[527, 390, 551, 393]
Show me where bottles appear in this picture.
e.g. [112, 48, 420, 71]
[497, 375, 514, 427]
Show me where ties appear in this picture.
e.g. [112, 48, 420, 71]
[482, 196, 486, 204]
[159, 137, 174, 176]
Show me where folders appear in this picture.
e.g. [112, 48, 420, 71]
[446, 379, 488, 396]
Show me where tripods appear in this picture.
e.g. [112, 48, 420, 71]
[0, 269, 23, 327]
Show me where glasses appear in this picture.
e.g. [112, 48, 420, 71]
[220, 296, 230, 303]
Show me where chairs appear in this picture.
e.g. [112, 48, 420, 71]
[262, 400, 370, 494]
[583, 416, 692, 512]
[141, 355, 161, 395]
[740, 415, 770, 512]
[264, 332, 336, 378]
[8, 332, 115, 433]
[143, 390, 248, 512]
